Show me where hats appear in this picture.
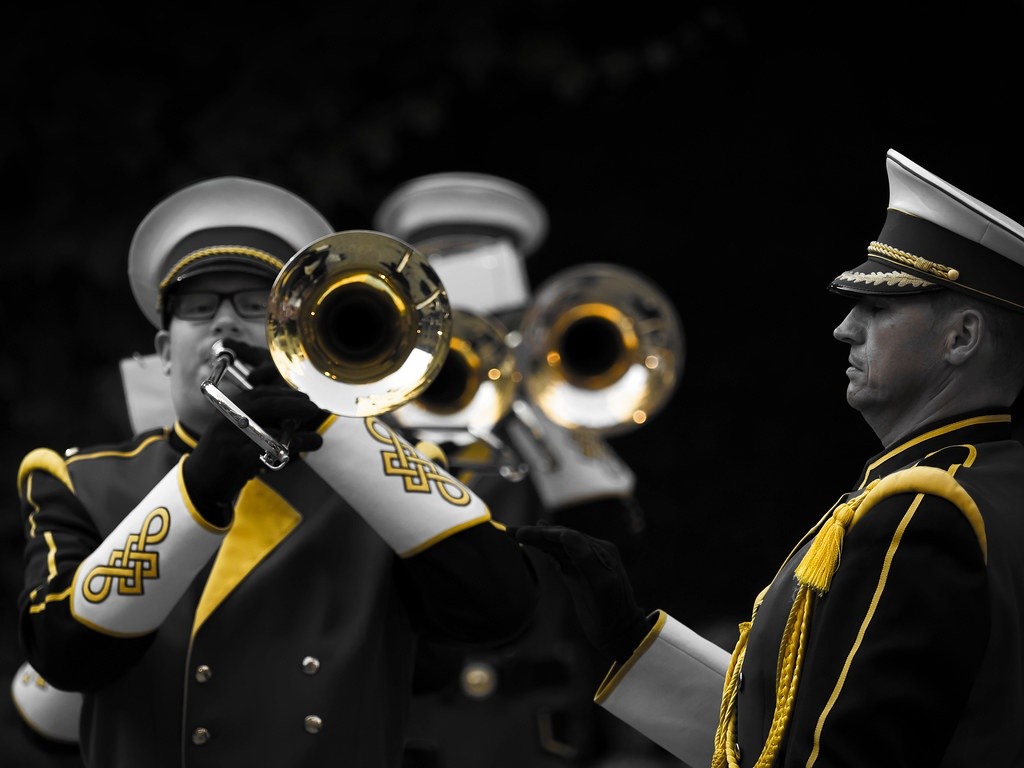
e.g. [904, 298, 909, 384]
[376, 173, 549, 262]
[827, 148, 1024, 307]
[127, 176, 338, 329]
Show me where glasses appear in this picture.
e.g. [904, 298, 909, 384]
[165, 285, 271, 321]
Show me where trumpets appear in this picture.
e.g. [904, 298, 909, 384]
[396, 304, 519, 452]
[524, 268, 689, 436]
[199, 225, 450, 456]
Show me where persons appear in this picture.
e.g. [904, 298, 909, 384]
[517, 148, 1024, 768]
[9, 175, 548, 768]
[374, 169, 636, 768]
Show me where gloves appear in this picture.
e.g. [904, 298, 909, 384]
[181, 360, 324, 528]
